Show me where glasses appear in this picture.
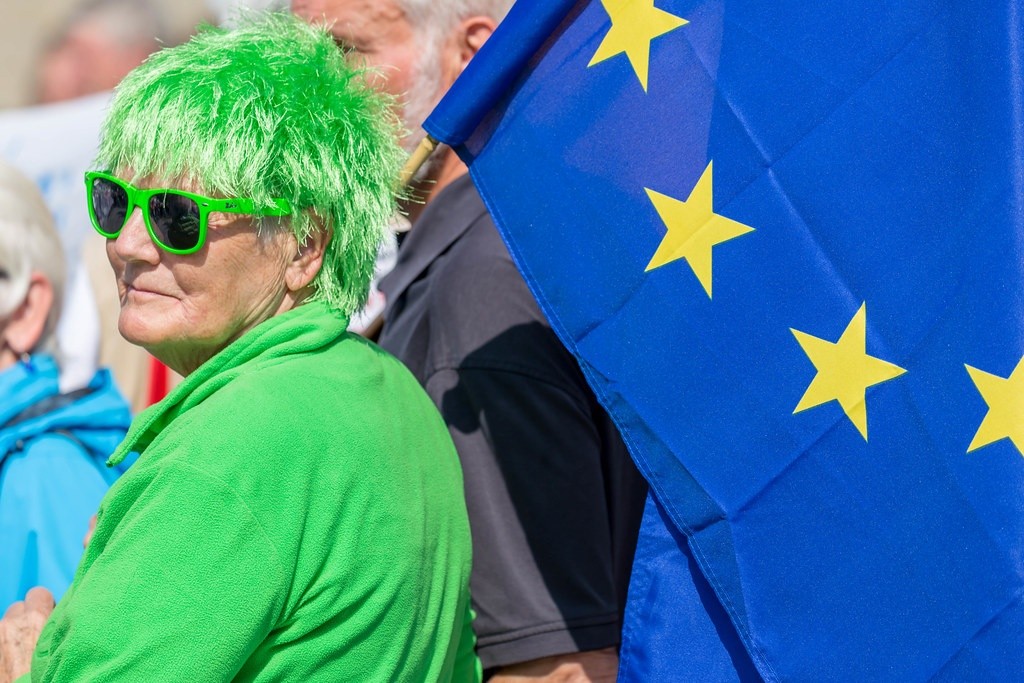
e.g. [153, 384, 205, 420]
[85, 170, 292, 254]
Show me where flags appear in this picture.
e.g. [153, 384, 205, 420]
[418, 1, 1024, 683]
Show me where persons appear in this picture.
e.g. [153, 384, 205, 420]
[31, 1, 219, 101]
[292, 0, 653, 681]
[2, 6, 486, 683]
[1, 154, 142, 632]
